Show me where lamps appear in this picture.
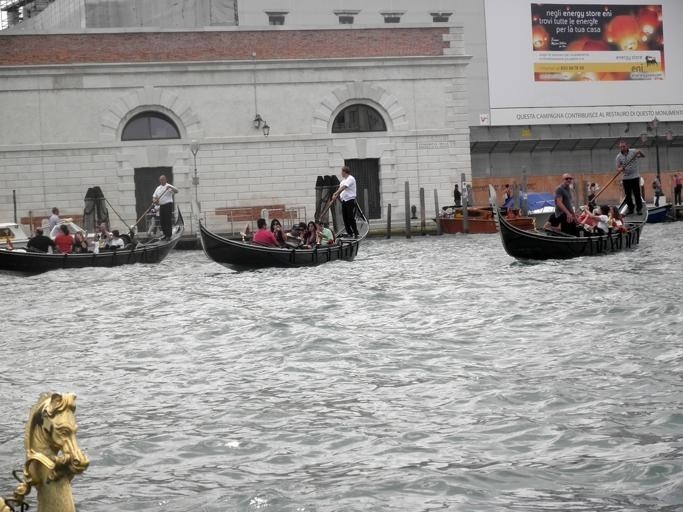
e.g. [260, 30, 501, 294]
[252, 52, 269, 136]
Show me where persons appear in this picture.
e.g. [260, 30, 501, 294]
[332, 167, 359, 238]
[503, 184, 510, 201]
[253, 219, 334, 247]
[153, 176, 178, 241]
[454, 184, 462, 205]
[673, 175, 683, 205]
[151, 203, 163, 234]
[544, 173, 624, 236]
[655, 176, 664, 206]
[26, 208, 124, 253]
[616, 142, 645, 215]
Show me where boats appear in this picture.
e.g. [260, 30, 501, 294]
[0, 206, 186, 271]
[195, 220, 370, 273]
[431, 191, 683, 262]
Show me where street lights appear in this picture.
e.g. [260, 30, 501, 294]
[189, 140, 199, 237]
[640, 117, 674, 206]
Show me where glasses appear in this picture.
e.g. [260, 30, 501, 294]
[565, 178, 572, 181]
